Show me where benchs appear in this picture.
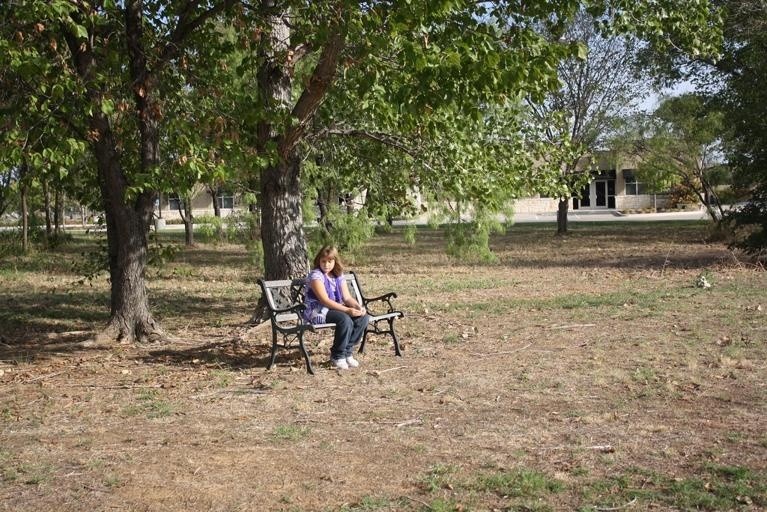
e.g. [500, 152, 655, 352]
[256, 270, 404, 376]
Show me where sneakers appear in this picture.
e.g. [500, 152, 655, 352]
[330, 356, 348, 369]
[346, 356, 359, 367]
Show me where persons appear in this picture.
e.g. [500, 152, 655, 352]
[303, 245, 368, 369]
